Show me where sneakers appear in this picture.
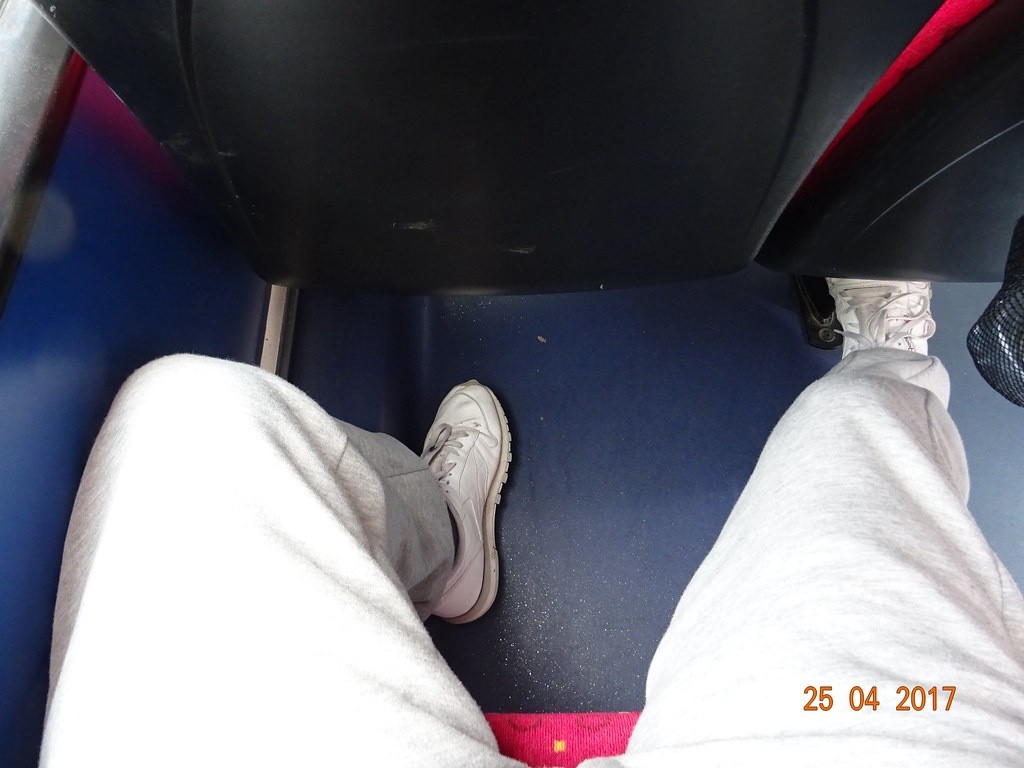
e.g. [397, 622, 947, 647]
[417, 381, 512, 625]
[825, 276, 936, 358]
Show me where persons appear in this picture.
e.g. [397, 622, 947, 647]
[38, 278, 1024, 768]
